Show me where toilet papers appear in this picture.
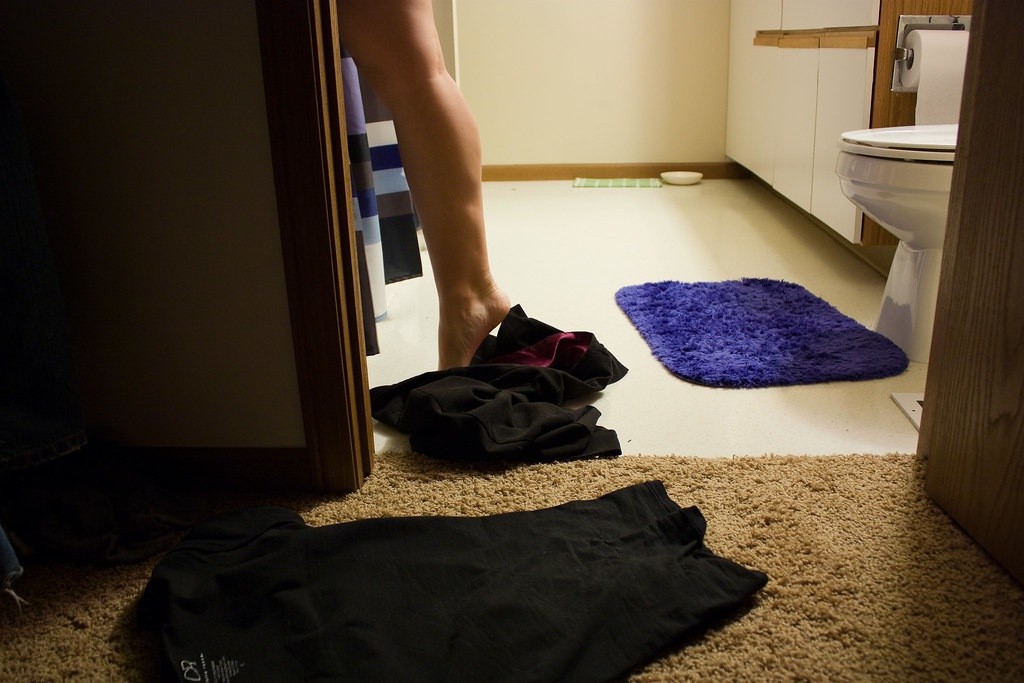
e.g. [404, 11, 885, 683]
[899, 29, 970, 126]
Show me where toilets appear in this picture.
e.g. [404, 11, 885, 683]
[834, 123, 959, 365]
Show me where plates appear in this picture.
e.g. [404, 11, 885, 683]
[660, 170, 703, 185]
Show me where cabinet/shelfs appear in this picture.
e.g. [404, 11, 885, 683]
[725, 0, 972, 248]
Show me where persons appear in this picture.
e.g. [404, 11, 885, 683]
[336, 0, 510, 371]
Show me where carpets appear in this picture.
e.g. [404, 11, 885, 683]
[615, 278, 910, 390]
[0, 455, 1024, 683]
